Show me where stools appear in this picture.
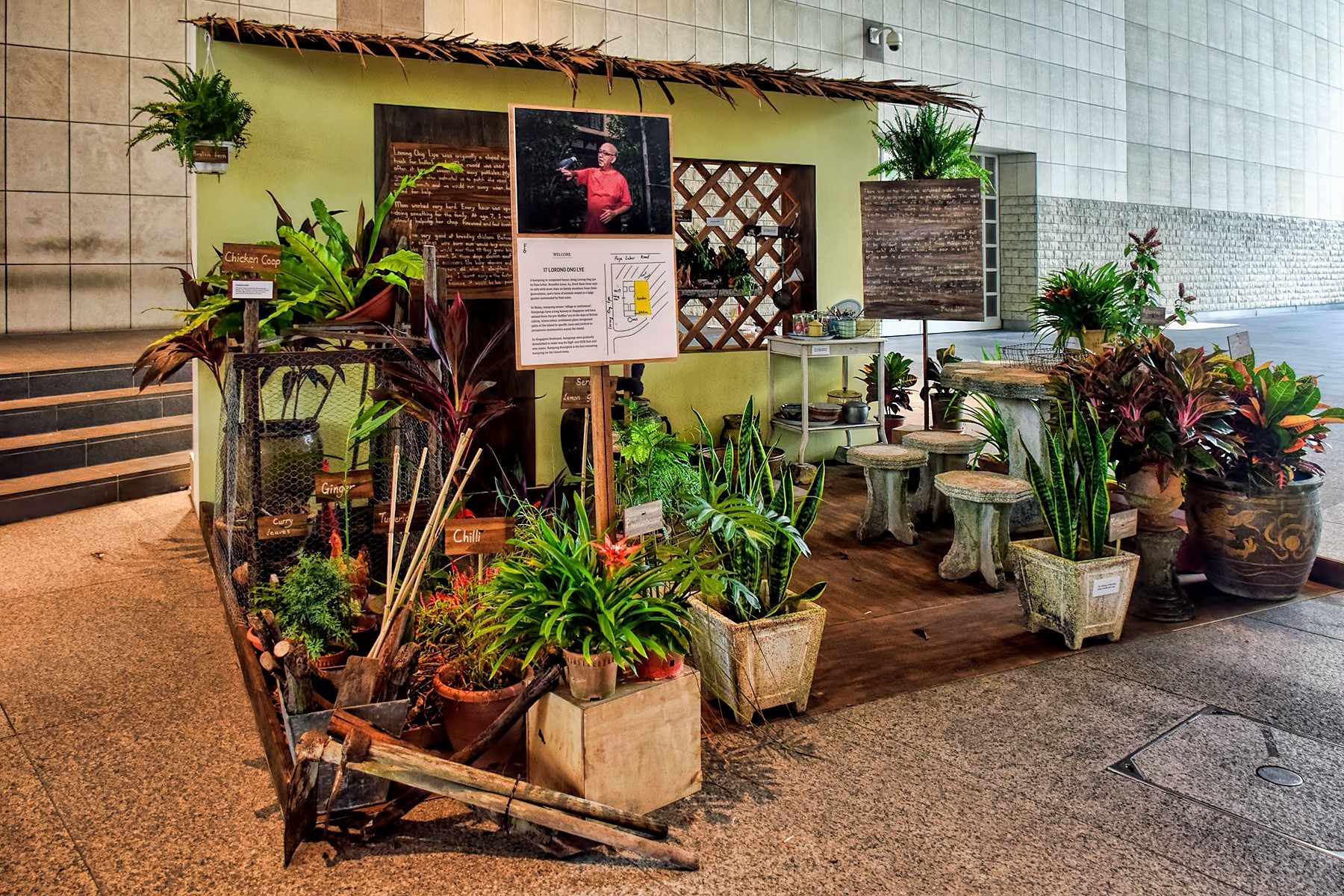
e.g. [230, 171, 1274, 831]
[934, 470, 1035, 589]
[902, 431, 988, 523]
[844, 443, 928, 544]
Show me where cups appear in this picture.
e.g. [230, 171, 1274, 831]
[792, 310, 811, 336]
[804, 318, 823, 336]
[838, 320, 856, 338]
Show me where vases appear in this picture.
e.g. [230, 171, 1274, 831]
[697, 443, 787, 496]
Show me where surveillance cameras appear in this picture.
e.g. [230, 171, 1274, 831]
[885, 31, 903, 51]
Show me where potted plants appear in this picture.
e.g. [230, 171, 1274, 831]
[131, 175, 830, 833]
[124, 62, 257, 175]
[854, 224, 1344, 652]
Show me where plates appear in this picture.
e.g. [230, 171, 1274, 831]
[826, 299, 862, 316]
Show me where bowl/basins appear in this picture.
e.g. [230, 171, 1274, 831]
[809, 403, 842, 421]
[779, 404, 802, 421]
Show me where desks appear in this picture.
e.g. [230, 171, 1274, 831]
[939, 360, 1066, 532]
[763, 334, 888, 465]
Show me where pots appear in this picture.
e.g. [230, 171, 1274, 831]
[827, 387, 862, 422]
[842, 402, 868, 424]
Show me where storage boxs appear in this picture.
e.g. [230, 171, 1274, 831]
[891, 425, 925, 445]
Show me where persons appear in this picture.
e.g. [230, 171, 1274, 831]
[561, 142, 634, 235]
